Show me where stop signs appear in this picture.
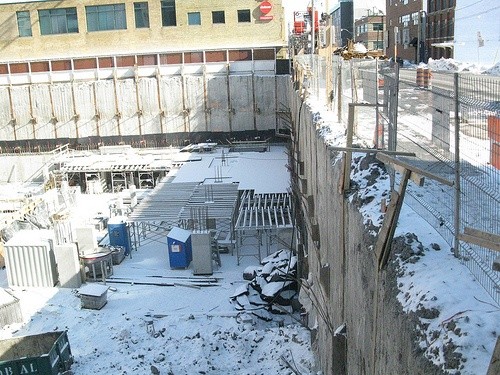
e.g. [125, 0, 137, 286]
[259, 1, 272, 14]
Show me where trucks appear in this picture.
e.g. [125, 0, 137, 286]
[0, 330, 74, 375]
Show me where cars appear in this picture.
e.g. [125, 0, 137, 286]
[379, 56, 404, 69]
